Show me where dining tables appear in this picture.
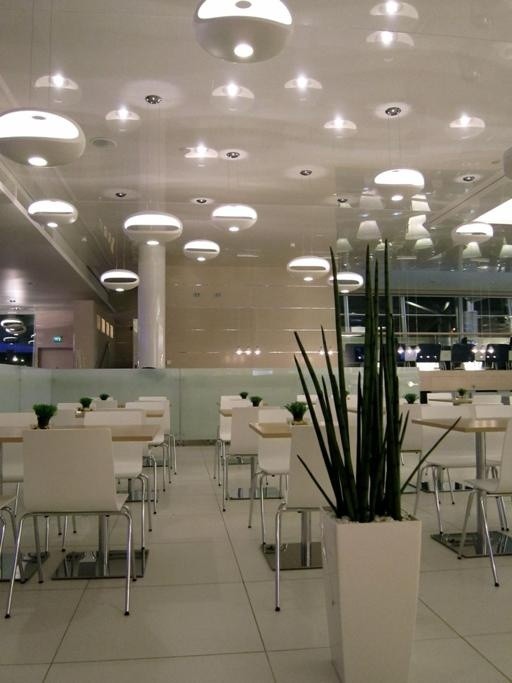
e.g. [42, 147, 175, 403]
[292, 234, 466, 683]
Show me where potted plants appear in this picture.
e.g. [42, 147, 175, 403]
[292, 234, 466, 683]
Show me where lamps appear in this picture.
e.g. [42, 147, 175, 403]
[1, 0, 512, 295]
[345, 336, 511, 370]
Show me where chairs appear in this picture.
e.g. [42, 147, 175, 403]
[345, 336, 511, 370]
[1, 383, 511, 619]
